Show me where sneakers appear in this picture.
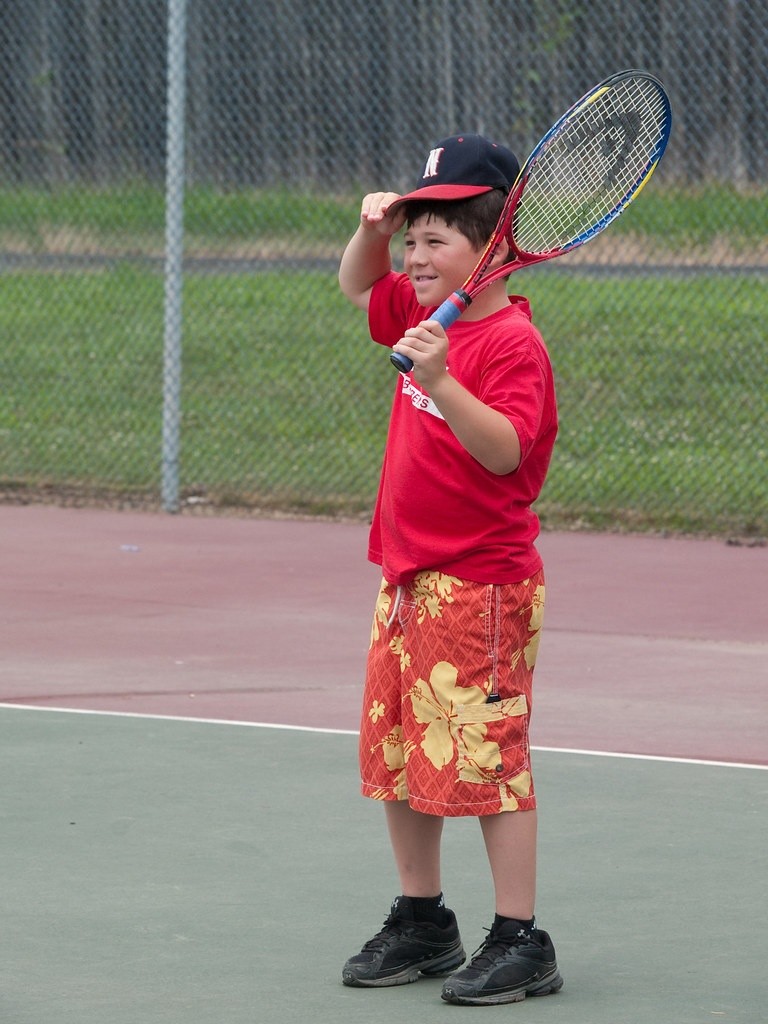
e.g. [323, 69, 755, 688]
[342, 896, 466, 987]
[441, 920, 564, 1006]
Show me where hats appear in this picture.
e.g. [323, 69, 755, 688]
[385, 134, 521, 218]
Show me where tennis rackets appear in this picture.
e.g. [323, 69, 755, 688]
[391, 70, 672, 372]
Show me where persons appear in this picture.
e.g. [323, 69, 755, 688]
[338, 134, 564, 1007]
